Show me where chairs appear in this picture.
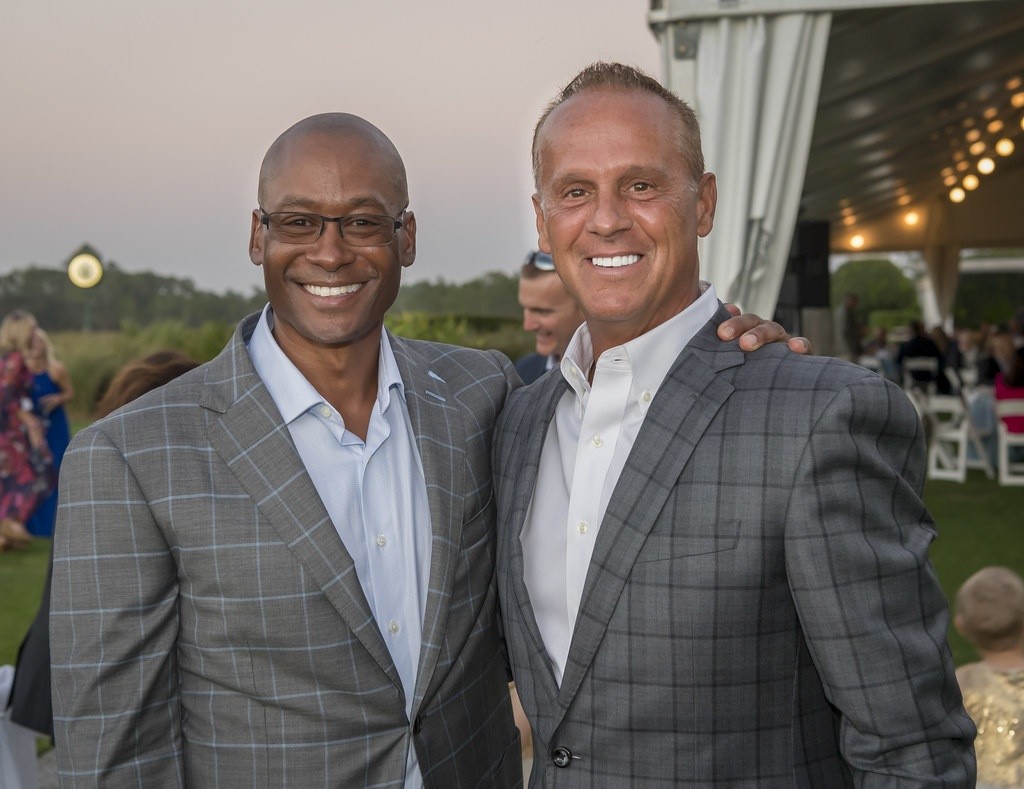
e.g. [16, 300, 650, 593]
[994, 398, 1024, 487]
[909, 385, 995, 483]
[902, 356, 938, 396]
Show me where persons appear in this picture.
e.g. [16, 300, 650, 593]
[56, 114, 810, 789]
[519, 252, 591, 386]
[4, 352, 206, 751]
[0, 310, 76, 560]
[844, 287, 1024, 463]
[952, 565, 1024, 789]
[487, 64, 978, 789]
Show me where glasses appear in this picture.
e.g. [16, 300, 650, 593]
[521, 250, 556, 273]
[259, 201, 409, 248]
